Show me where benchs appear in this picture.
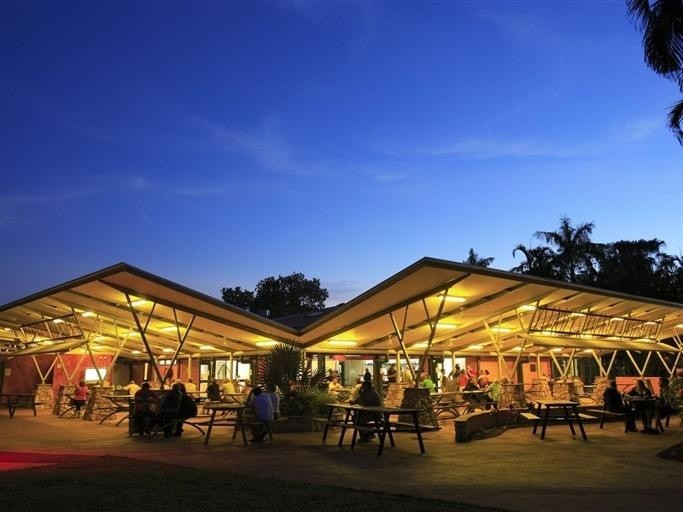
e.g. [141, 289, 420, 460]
[0, 392, 45, 418]
[434, 394, 498, 420]
[520, 392, 672, 441]
[312, 402, 438, 457]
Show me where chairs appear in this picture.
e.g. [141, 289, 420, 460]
[57, 394, 134, 427]
[185, 392, 287, 446]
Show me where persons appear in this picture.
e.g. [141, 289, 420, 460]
[174, 382, 198, 437]
[146, 383, 183, 440]
[247, 387, 275, 443]
[604, 380, 639, 432]
[329, 375, 345, 396]
[626, 378, 653, 433]
[70, 379, 88, 418]
[646, 377, 678, 435]
[363, 367, 373, 381]
[348, 378, 383, 444]
[387, 363, 581, 410]
[95, 377, 252, 417]
[344, 375, 362, 402]
[132, 382, 160, 440]
[260, 384, 281, 442]
